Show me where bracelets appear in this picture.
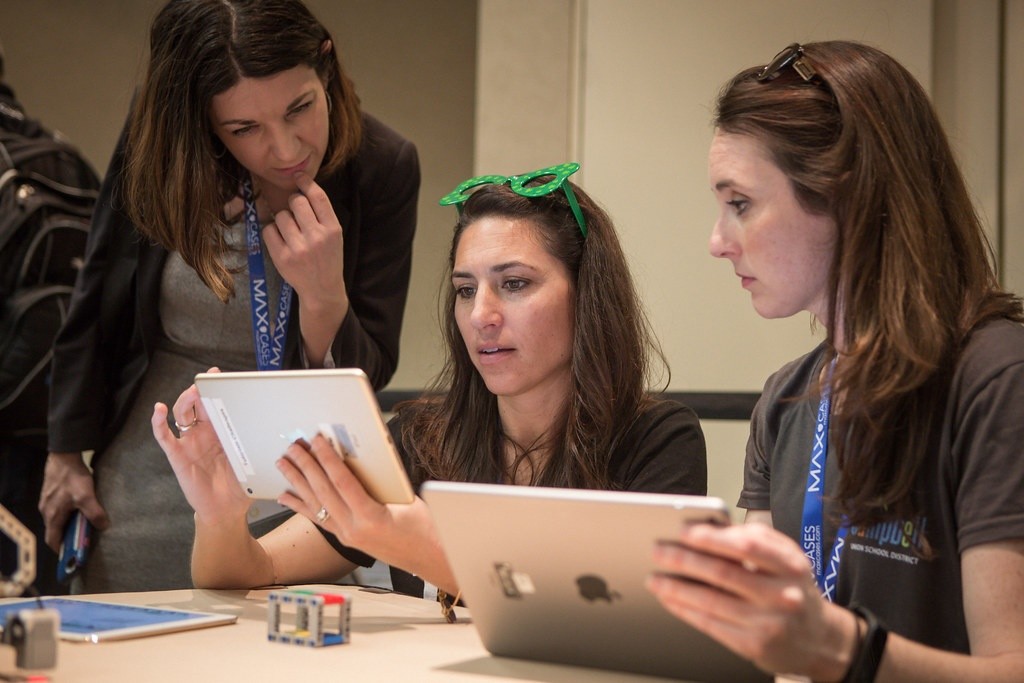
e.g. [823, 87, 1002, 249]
[437, 586, 463, 623]
[839, 601, 889, 683]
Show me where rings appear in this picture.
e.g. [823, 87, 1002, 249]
[175, 418, 197, 432]
[315, 507, 331, 524]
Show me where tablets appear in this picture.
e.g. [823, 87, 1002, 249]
[421, 481, 812, 683]
[0, 596, 238, 643]
[195, 369, 415, 505]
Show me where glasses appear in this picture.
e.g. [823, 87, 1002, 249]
[755, 43, 819, 85]
[437, 162, 588, 238]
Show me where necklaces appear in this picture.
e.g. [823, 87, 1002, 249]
[801, 356, 846, 604]
[236, 169, 294, 369]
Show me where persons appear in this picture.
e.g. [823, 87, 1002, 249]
[40, 0, 424, 598]
[0, 54, 104, 594]
[147, 171, 709, 601]
[642, 40, 1024, 683]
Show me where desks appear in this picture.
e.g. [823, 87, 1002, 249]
[0, 583, 811, 683]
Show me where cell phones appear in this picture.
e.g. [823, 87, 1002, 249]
[56, 509, 92, 584]
